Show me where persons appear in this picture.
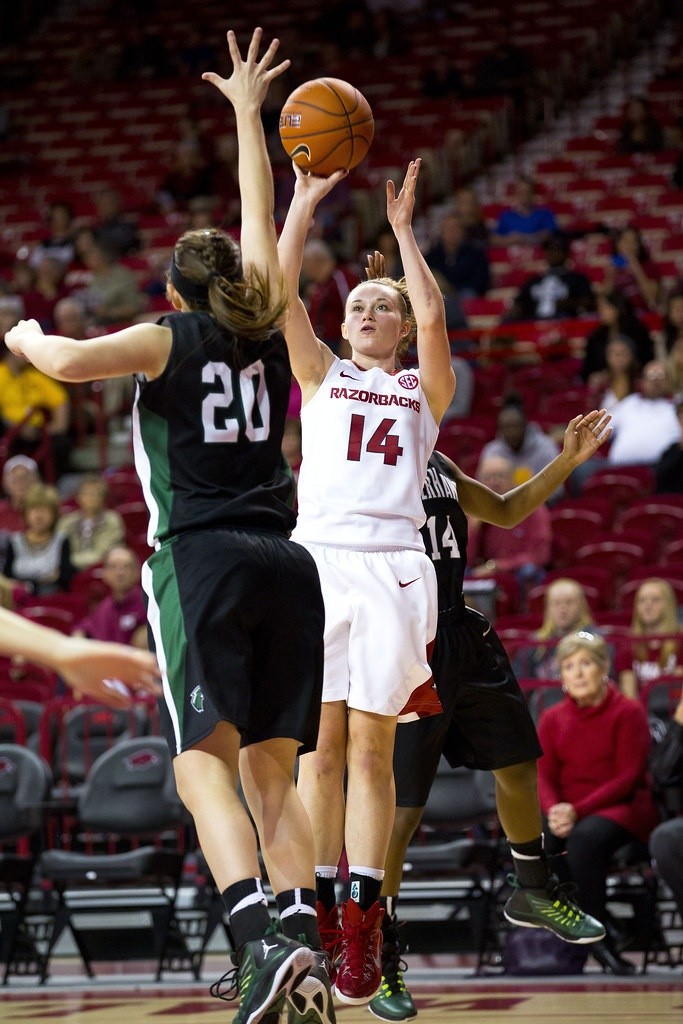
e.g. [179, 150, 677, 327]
[276, 157, 456, 1005]
[0, 176, 683, 976]
[371, 407, 606, 1024]
[3, 24, 333, 1023]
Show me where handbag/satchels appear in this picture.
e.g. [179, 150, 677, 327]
[497, 926, 589, 975]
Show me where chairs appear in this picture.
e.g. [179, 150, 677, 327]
[192, 786, 278, 980]
[37, 735, 199, 986]
[0, 743, 54, 985]
[404, 757, 498, 978]
[0, 0, 683, 886]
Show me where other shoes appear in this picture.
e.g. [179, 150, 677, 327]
[593, 947, 635, 974]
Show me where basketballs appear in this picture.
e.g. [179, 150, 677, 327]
[278, 76, 375, 175]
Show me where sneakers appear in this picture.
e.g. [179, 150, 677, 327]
[368, 914, 417, 1023]
[316, 900, 385, 1005]
[503, 887, 606, 944]
[210, 917, 336, 1024]
